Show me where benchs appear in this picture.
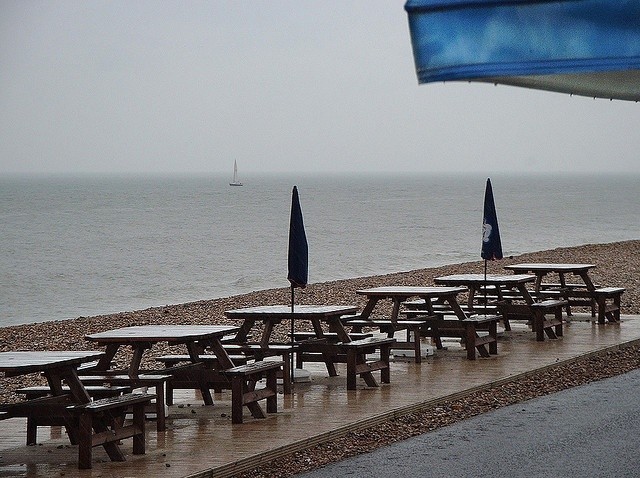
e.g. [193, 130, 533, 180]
[428, 306, 498, 316]
[532, 301, 568, 340]
[594, 286, 626, 326]
[65, 396, 157, 468]
[487, 290, 562, 300]
[76, 373, 172, 433]
[343, 337, 396, 389]
[288, 329, 373, 376]
[474, 294, 536, 332]
[154, 353, 246, 404]
[340, 312, 362, 325]
[191, 334, 252, 346]
[347, 318, 427, 364]
[220, 343, 299, 387]
[13, 385, 130, 447]
[543, 283, 600, 318]
[404, 299, 439, 341]
[400, 310, 476, 342]
[463, 315, 504, 362]
[224, 361, 286, 424]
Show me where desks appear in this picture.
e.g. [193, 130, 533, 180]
[224, 305, 378, 387]
[355, 285, 490, 357]
[85, 324, 268, 420]
[433, 273, 537, 330]
[504, 261, 595, 319]
[0, 349, 125, 460]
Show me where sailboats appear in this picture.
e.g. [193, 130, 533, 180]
[229, 159, 243, 186]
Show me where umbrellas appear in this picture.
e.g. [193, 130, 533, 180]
[480, 177, 503, 313]
[288, 185, 309, 382]
